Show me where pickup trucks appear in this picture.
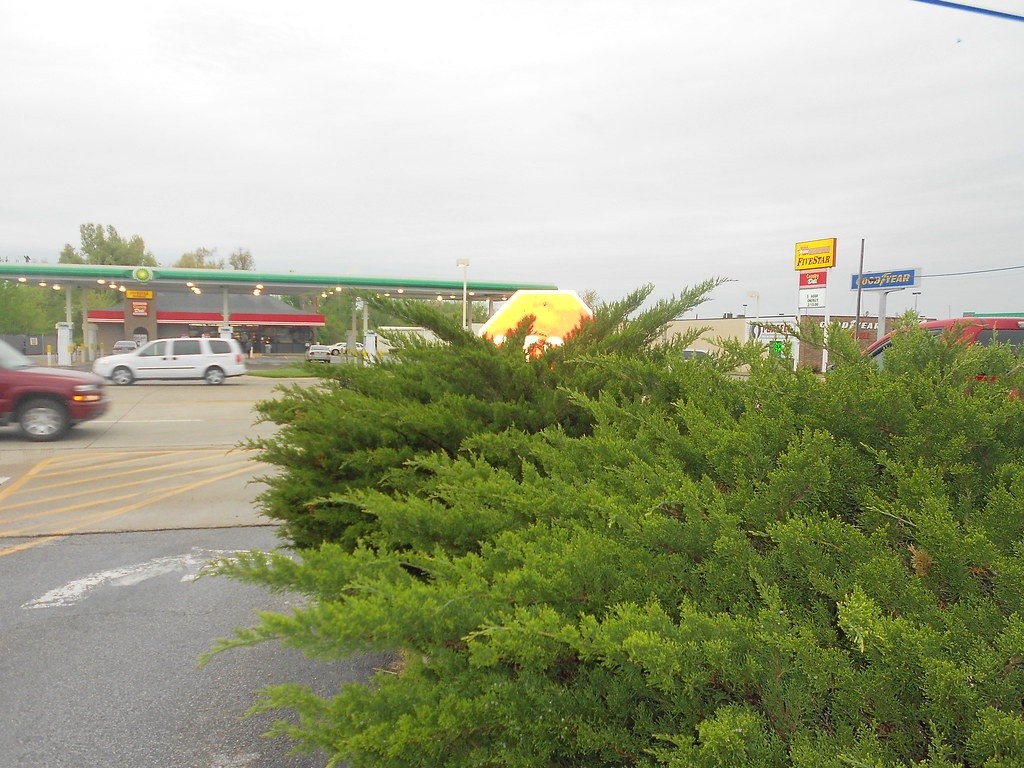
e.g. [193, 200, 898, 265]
[827, 317, 1024, 430]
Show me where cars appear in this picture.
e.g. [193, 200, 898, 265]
[113, 341, 137, 355]
[683, 348, 709, 358]
[0, 339, 108, 442]
[306, 344, 331, 363]
[326, 342, 363, 356]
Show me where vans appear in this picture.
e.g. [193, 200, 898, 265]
[93, 336, 247, 385]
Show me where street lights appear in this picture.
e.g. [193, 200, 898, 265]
[457, 259, 470, 329]
[913, 292, 921, 317]
